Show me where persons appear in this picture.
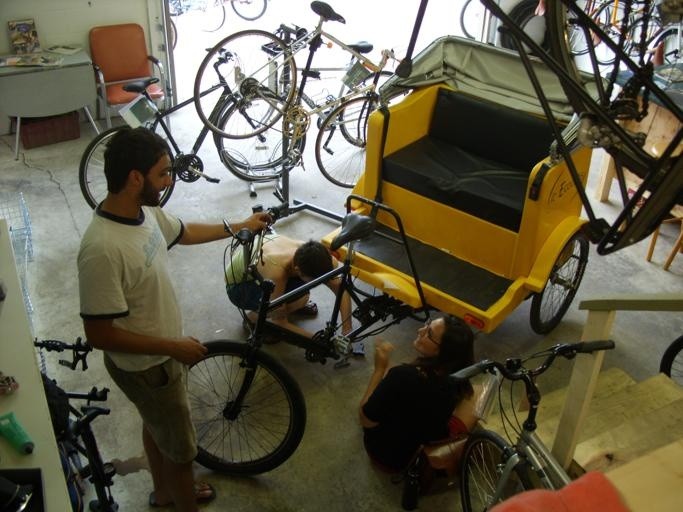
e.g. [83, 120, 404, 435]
[356, 312, 473, 493]
[77, 125, 272, 512]
[223, 234, 352, 340]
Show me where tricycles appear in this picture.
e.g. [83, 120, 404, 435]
[184, 33, 591, 479]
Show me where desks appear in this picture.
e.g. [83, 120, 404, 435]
[598, 60, 683, 233]
[0, 45, 106, 165]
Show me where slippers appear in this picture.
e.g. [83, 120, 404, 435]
[149, 480, 217, 507]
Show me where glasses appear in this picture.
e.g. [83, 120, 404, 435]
[423, 318, 440, 347]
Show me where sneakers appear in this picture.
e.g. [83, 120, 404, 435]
[296, 301, 318, 316]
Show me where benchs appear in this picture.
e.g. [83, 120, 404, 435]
[381, 81, 576, 234]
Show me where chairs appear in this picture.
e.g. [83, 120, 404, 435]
[88, 23, 175, 141]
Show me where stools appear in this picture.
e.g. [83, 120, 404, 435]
[644, 198, 683, 274]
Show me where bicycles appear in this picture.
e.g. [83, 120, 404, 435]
[444, 334, 615, 512]
[461, 0, 683, 75]
[658, 333, 681, 385]
